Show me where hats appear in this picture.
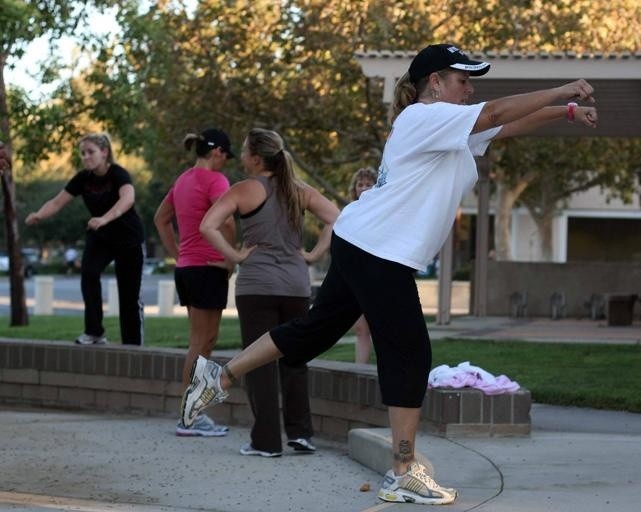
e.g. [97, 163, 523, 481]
[409, 44, 490, 83]
[200, 129, 235, 159]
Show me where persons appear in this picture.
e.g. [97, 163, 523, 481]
[179, 41, 599, 506]
[347, 166, 378, 364]
[64, 245, 79, 277]
[198, 128, 342, 459]
[25, 133, 147, 347]
[152, 128, 239, 437]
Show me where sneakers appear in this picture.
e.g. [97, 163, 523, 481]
[240, 444, 283, 457]
[377, 461, 457, 505]
[180, 355, 230, 429]
[75, 333, 107, 345]
[287, 438, 316, 451]
[176, 414, 229, 437]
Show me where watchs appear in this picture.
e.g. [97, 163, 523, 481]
[567, 102, 578, 123]
